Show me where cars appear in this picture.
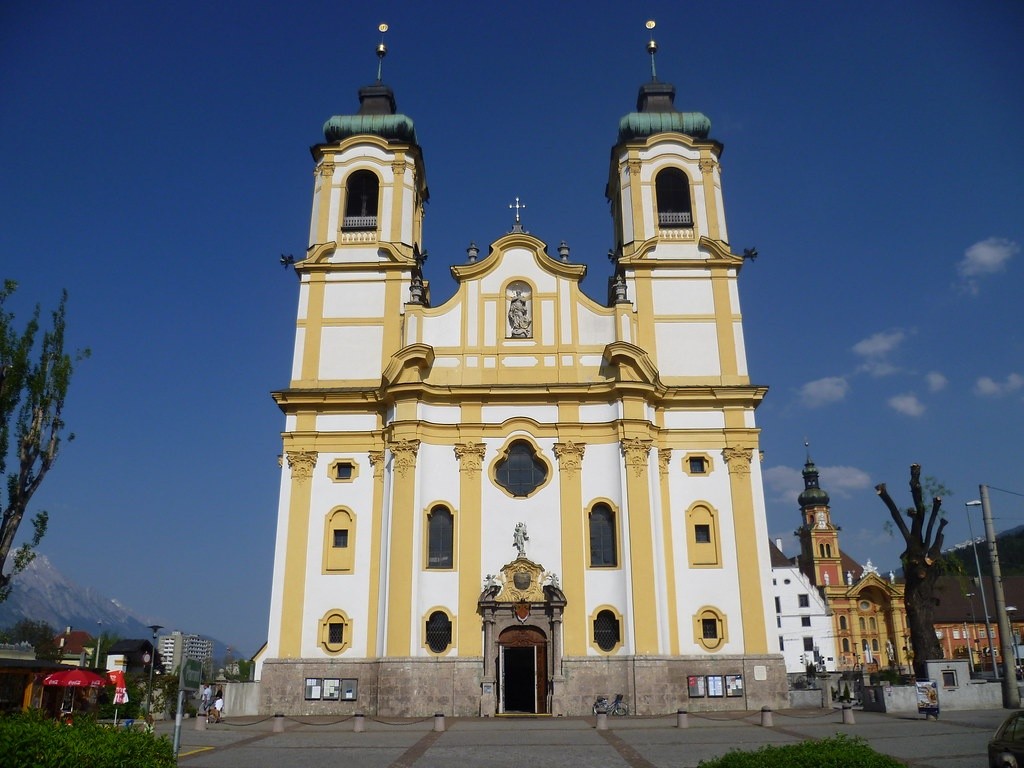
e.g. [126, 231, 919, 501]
[988, 710, 1024, 768]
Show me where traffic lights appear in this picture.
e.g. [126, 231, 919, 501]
[800, 654, 806, 665]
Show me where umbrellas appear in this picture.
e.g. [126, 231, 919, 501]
[44, 669, 107, 687]
[108, 671, 129, 705]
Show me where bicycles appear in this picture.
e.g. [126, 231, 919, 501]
[592, 693, 628, 716]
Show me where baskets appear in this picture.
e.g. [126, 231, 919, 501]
[617, 695, 623, 701]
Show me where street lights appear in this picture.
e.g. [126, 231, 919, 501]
[144, 625, 165, 721]
[965, 499, 1001, 682]
[95, 619, 101, 668]
[966, 592, 984, 673]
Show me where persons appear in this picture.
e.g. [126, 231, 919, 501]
[199, 684, 223, 724]
[142, 714, 158, 736]
[34, 693, 54, 717]
[60, 693, 72, 719]
